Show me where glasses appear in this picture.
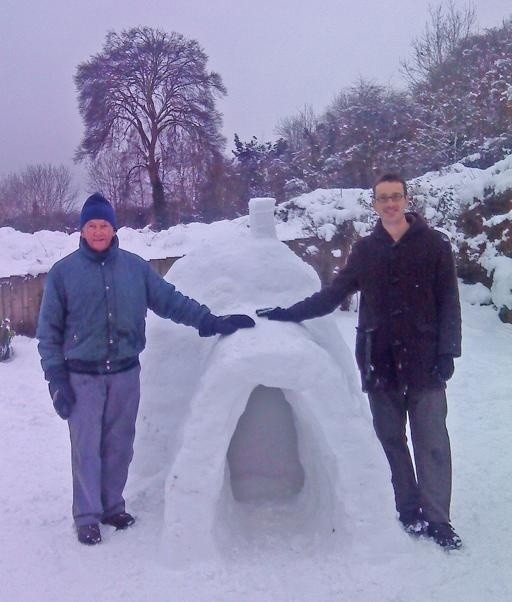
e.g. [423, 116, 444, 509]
[373, 192, 404, 204]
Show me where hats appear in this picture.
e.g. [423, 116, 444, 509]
[80, 191, 118, 233]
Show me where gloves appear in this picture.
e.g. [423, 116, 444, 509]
[255, 307, 295, 323]
[48, 372, 77, 420]
[199, 312, 256, 337]
[430, 354, 455, 385]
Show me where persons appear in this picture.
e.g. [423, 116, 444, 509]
[37, 192, 257, 544]
[251, 173, 467, 552]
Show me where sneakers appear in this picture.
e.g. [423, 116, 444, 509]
[102, 511, 136, 528]
[427, 525, 463, 550]
[400, 509, 425, 539]
[75, 525, 102, 544]
